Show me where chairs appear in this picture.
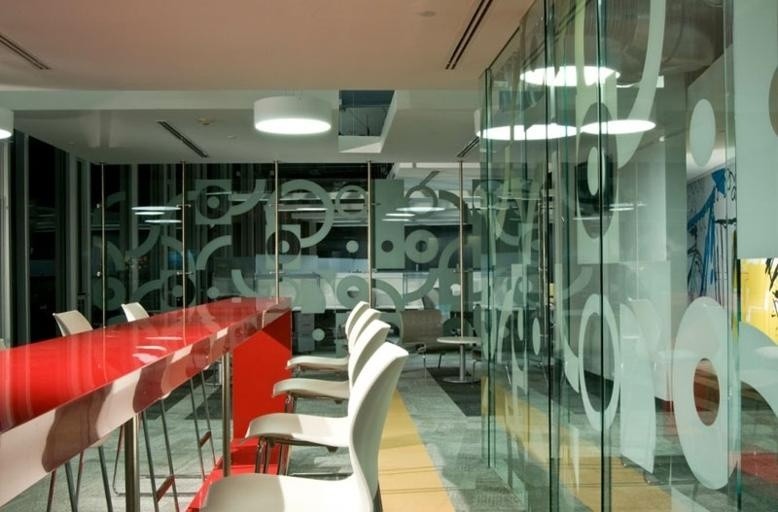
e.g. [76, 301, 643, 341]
[46, 310, 180, 512]
[272, 308, 382, 474]
[112, 301, 216, 496]
[1, 338, 113, 511]
[284, 301, 370, 477]
[200, 342, 409, 512]
[398, 310, 473, 383]
[244, 318, 391, 474]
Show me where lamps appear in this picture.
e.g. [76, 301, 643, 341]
[253, 96, 332, 135]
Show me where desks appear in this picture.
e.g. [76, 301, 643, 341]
[0, 298, 292, 512]
